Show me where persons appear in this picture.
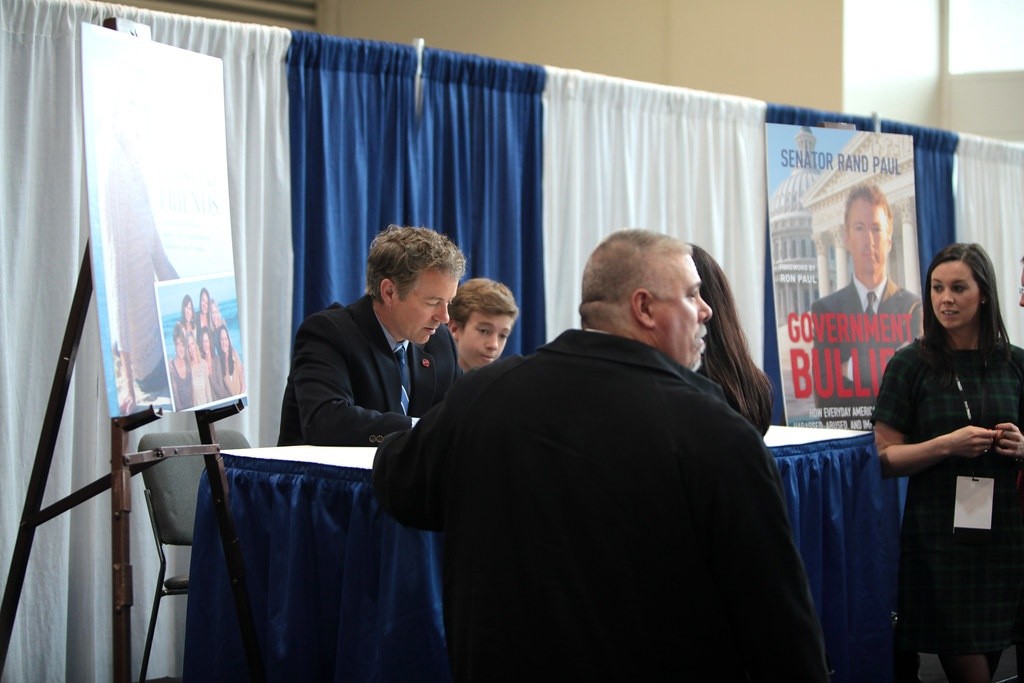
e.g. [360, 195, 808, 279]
[1019, 255, 1024, 307]
[684, 242, 774, 439]
[869, 242, 1024, 683]
[372, 228, 823, 683]
[447, 277, 519, 374]
[811, 181, 924, 431]
[104, 136, 246, 415]
[275, 224, 466, 446]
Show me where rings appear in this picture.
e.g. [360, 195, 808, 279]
[984, 445, 988, 453]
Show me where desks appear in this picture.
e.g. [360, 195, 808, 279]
[182, 425, 900, 683]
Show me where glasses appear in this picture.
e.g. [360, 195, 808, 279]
[1019, 286, 1024, 295]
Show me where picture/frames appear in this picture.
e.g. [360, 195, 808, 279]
[154, 269, 249, 413]
[764, 122, 925, 431]
[81, 21, 235, 419]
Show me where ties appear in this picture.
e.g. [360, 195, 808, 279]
[866, 292, 876, 323]
[393, 343, 410, 416]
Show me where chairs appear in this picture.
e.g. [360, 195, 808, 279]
[137, 429, 251, 683]
